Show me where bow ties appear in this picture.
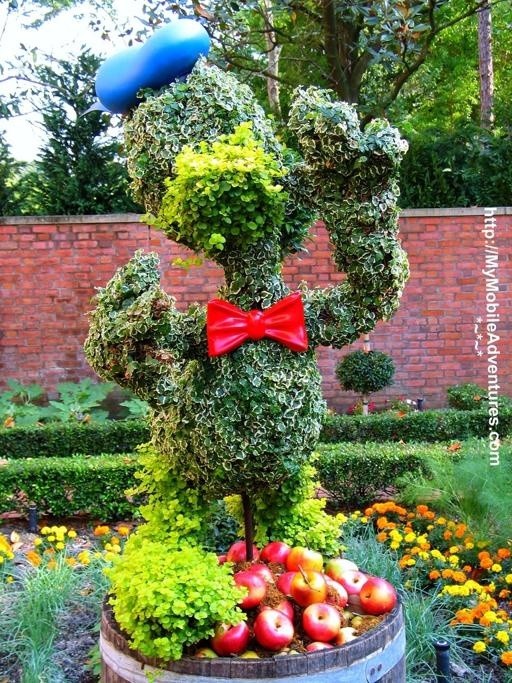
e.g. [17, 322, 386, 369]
[202, 293, 310, 358]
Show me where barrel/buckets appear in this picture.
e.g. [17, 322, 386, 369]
[99, 570, 406, 683]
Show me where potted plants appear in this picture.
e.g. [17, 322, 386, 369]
[90, 14, 410, 683]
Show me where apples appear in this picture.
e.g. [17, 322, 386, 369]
[190, 541, 398, 660]
